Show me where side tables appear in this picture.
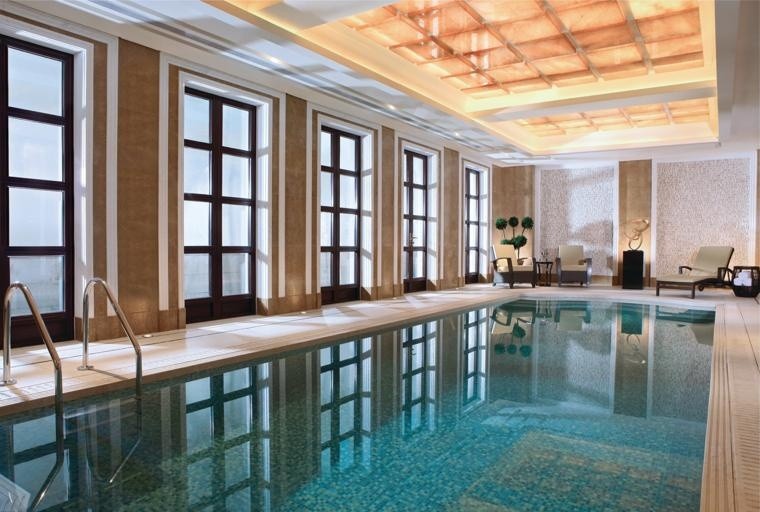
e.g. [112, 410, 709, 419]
[535, 261, 554, 287]
[536, 300, 553, 318]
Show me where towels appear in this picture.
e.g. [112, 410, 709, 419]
[733, 271, 753, 287]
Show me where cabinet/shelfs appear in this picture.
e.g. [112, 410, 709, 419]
[731, 266, 760, 297]
[622, 250, 643, 289]
[621, 302, 644, 335]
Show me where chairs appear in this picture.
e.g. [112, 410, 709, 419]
[554, 300, 591, 331]
[555, 245, 593, 288]
[655, 246, 734, 299]
[489, 300, 536, 336]
[489, 243, 537, 289]
